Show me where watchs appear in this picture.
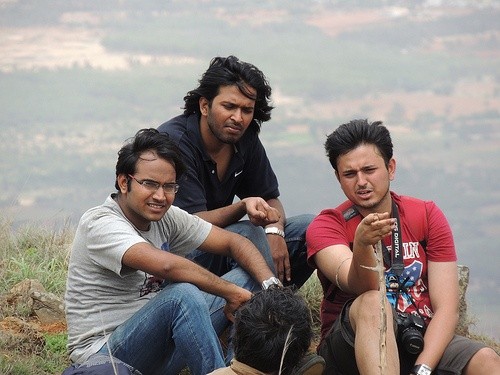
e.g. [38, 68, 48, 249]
[409, 364, 431, 375]
[261, 276, 280, 290]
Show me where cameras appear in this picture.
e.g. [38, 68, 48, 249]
[395, 311, 426, 354]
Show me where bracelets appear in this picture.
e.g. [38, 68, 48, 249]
[265, 227, 284, 237]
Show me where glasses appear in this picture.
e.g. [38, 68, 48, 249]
[128, 173, 180, 193]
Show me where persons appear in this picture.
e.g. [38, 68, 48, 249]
[205, 286, 314, 375]
[156, 55, 316, 293]
[65, 128, 283, 375]
[306, 118, 500, 375]
